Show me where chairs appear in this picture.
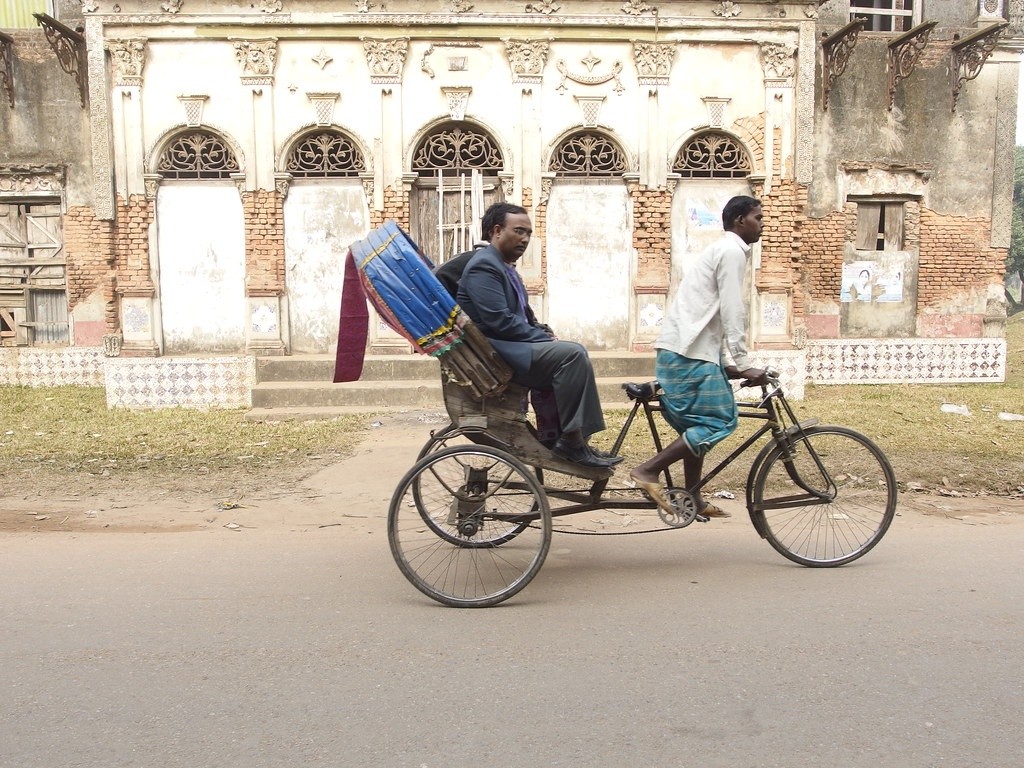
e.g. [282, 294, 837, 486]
[440, 351, 610, 481]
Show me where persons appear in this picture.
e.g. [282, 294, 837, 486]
[435, 202, 610, 457]
[456, 205, 625, 468]
[630, 195, 768, 517]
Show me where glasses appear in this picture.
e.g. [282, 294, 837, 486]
[496, 224, 532, 238]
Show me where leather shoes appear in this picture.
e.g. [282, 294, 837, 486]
[587, 445, 624, 466]
[552, 438, 612, 468]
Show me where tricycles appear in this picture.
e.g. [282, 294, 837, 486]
[334, 222, 897, 609]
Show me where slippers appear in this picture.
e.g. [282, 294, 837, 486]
[697, 502, 732, 518]
[632, 476, 673, 516]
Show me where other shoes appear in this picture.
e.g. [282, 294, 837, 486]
[540, 438, 557, 449]
